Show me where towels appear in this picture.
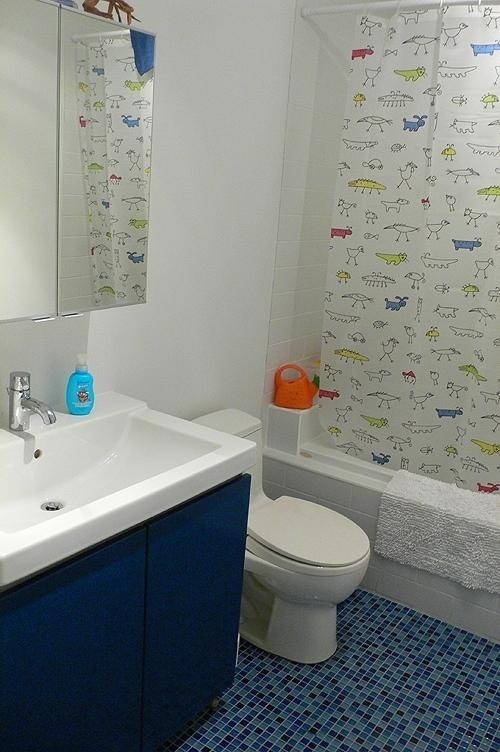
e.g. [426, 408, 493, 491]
[374, 469, 500, 595]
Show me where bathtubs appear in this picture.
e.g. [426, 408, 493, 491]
[305, 427, 498, 504]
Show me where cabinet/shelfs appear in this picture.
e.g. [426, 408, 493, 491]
[0, 474, 250, 752]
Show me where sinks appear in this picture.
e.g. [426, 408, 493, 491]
[0, 411, 223, 541]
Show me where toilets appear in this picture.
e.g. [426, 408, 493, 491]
[191, 409, 372, 664]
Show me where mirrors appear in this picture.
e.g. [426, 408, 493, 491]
[60, 2, 156, 311]
[0, 0, 60, 321]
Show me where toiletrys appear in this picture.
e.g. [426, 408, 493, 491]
[66, 353, 96, 416]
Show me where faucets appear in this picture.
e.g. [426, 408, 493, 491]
[6, 369, 58, 430]
